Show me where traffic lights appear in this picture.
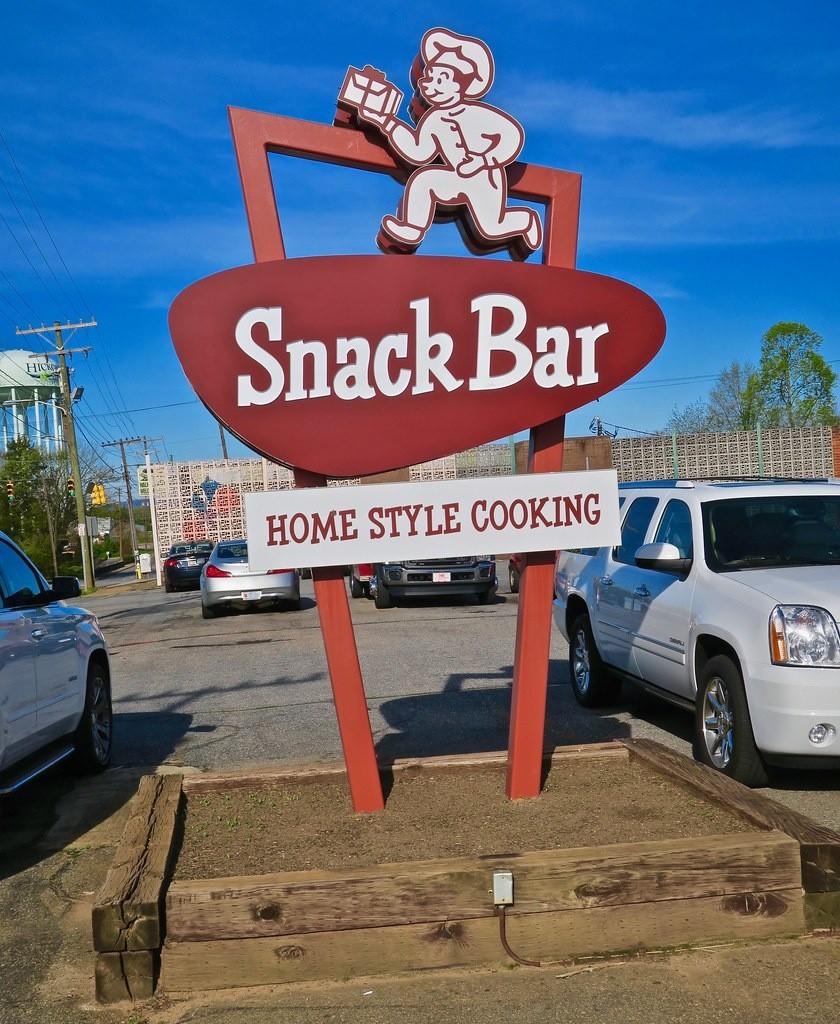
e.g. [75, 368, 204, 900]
[97, 485, 106, 504]
[7, 484, 15, 502]
[68, 478, 76, 496]
[91, 485, 101, 506]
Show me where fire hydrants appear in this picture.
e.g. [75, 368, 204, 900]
[134, 565, 143, 580]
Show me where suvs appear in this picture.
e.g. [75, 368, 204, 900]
[553, 474, 840, 790]
[0, 531, 116, 804]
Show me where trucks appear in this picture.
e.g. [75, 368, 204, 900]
[347, 553, 500, 609]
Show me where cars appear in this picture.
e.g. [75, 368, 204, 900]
[508, 548, 560, 592]
[200, 539, 302, 618]
[165, 539, 216, 593]
